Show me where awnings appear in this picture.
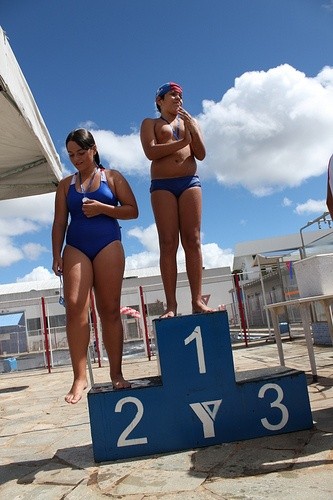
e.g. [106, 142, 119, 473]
[0, 311, 26, 329]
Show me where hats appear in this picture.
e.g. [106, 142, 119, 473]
[156, 82, 183, 97]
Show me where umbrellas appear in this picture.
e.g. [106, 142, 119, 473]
[1, 25, 64, 204]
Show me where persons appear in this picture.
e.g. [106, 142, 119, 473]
[49, 126, 138, 403]
[138, 81, 215, 319]
